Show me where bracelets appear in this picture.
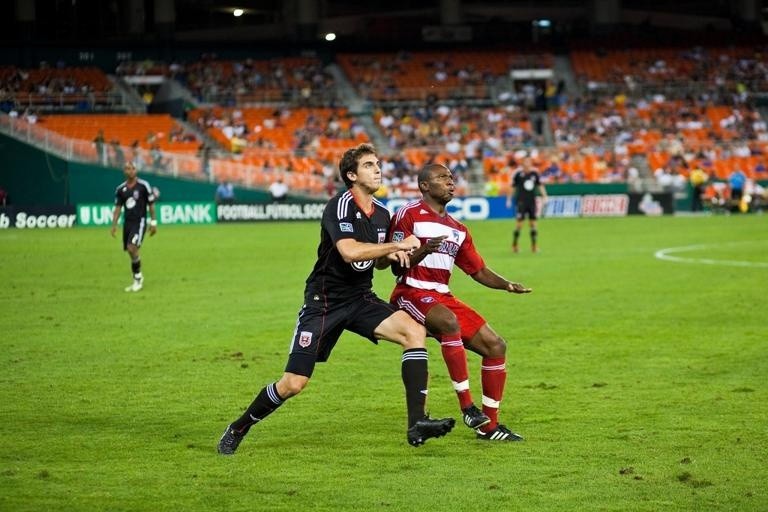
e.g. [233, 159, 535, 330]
[150, 220, 157, 226]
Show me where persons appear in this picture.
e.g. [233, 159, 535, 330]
[388, 162, 532, 441]
[507, 159, 547, 254]
[215, 143, 457, 453]
[111, 162, 158, 292]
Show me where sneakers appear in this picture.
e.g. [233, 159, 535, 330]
[124, 271, 145, 293]
[217, 424, 245, 455]
[406, 415, 457, 448]
[463, 404, 524, 441]
